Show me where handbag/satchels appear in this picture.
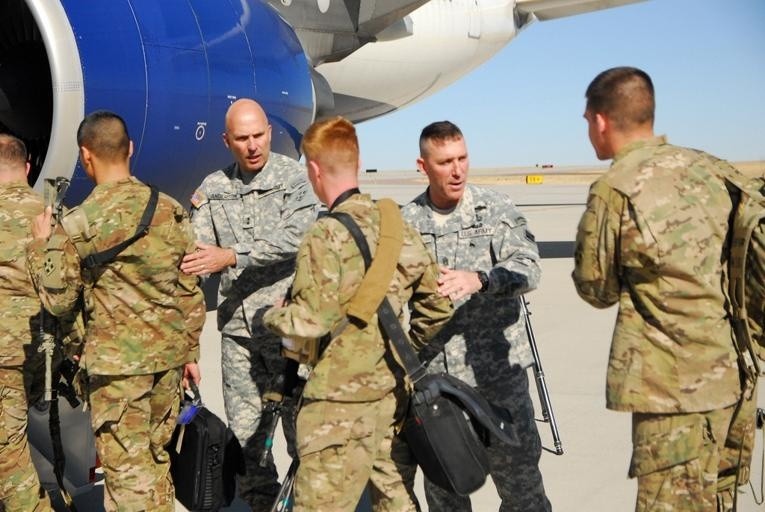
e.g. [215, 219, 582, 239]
[24, 336, 65, 410]
[411, 373, 520, 496]
[168, 394, 247, 511]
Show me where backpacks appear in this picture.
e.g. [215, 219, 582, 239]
[692, 149, 765, 384]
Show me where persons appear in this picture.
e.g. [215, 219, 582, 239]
[0, 135, 83, 511]
[385, 120, 553, 512]
[179, 96, 321, 512]
[25, 109, 207, 512]
[263, 117, 455, 512]
[569, 64, 757, 512]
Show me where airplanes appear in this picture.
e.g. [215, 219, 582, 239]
[0, 0, 649, 312]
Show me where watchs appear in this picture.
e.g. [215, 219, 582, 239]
[475, 269, 491, 294]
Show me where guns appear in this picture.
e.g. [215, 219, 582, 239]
[260, 286, 298, 469]
[38, 176, 62, 401]
